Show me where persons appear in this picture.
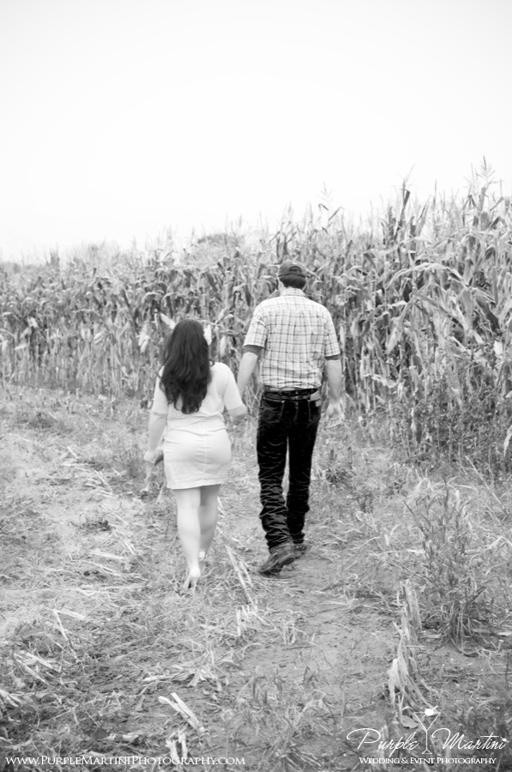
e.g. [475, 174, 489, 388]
[141, 317, 248, 595]
[234, 258, 348, 576]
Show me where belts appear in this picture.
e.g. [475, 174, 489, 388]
[263, 390, 315, 402]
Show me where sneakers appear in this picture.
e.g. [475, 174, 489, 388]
[293, 542, 307, 559]
[258, 535, 296, 577]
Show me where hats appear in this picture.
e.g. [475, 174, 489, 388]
[278, 259, 306, 278]
